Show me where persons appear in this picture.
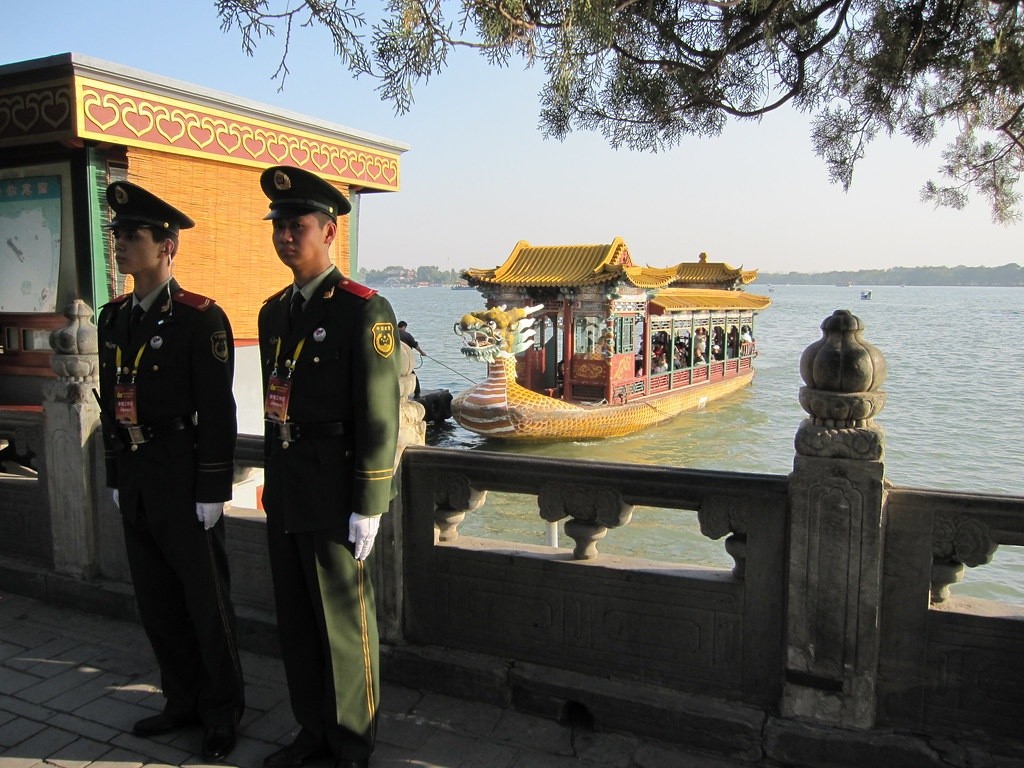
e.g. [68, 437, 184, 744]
[97, 181, 247, 764]
[598, 328, 607, 344]
[259, 165, 402, 768]
[636, 325, 755, 377]
[397, 321, 426, 399]
[581, 331, 593, 352]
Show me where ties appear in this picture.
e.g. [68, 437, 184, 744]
[128, 304, 145, 348]
[290, 291, 306, 336]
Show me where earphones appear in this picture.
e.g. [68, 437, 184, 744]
[161, 242, 167, 251]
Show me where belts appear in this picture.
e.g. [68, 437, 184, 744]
[267, 418, 343, 442]
[112, 411, 196, 444]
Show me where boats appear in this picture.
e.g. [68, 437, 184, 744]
[451, 281, 479, 290]
[450, 239, 772, 441]
[861, 289, 871, 300]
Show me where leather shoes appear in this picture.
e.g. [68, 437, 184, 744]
[202, 723, 236, 762]
[334, 758, 368, 768]
[263, 741, 327, 768]
[132, 712, 184, 737]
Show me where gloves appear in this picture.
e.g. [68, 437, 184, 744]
[348, 512, 382, 562]
[196, 502, 223, 530]
[112, 489, 120, 510]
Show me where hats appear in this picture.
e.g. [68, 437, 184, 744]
[103, 181, 195, 237]
[261, 166, 352, 221]
